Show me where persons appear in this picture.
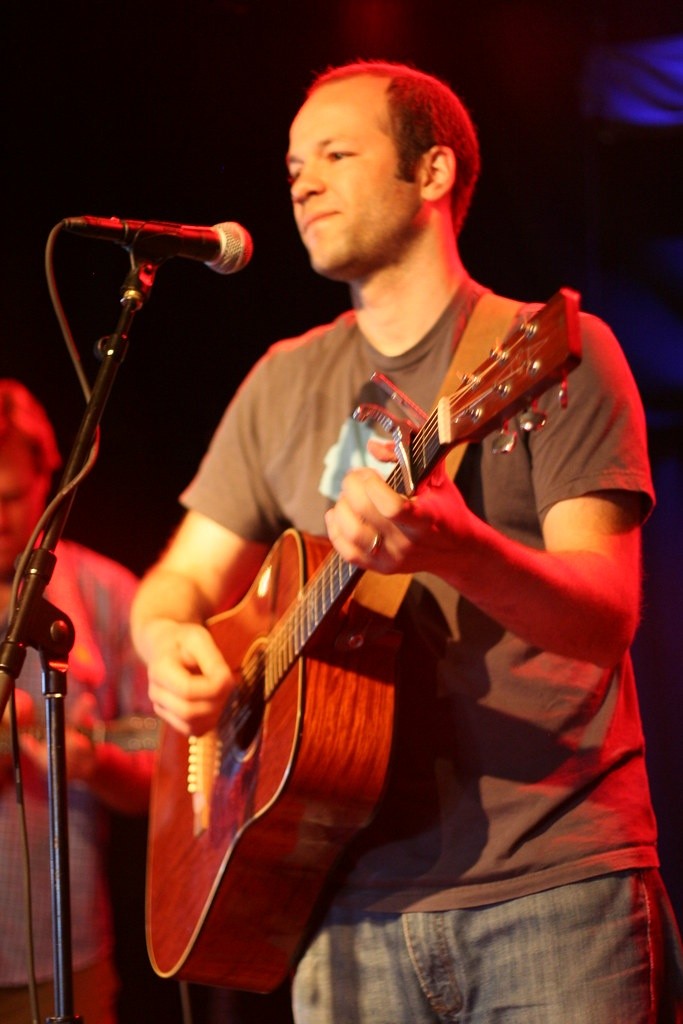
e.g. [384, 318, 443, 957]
[0, 377, 143, 1022]
[130, 63, 683, 1023]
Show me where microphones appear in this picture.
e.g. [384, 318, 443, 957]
[62, 215, 254, 274]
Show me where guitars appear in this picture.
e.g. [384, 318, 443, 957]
[141, 285, 584, 992]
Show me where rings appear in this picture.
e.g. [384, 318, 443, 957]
[365, 531, 383, 556]
